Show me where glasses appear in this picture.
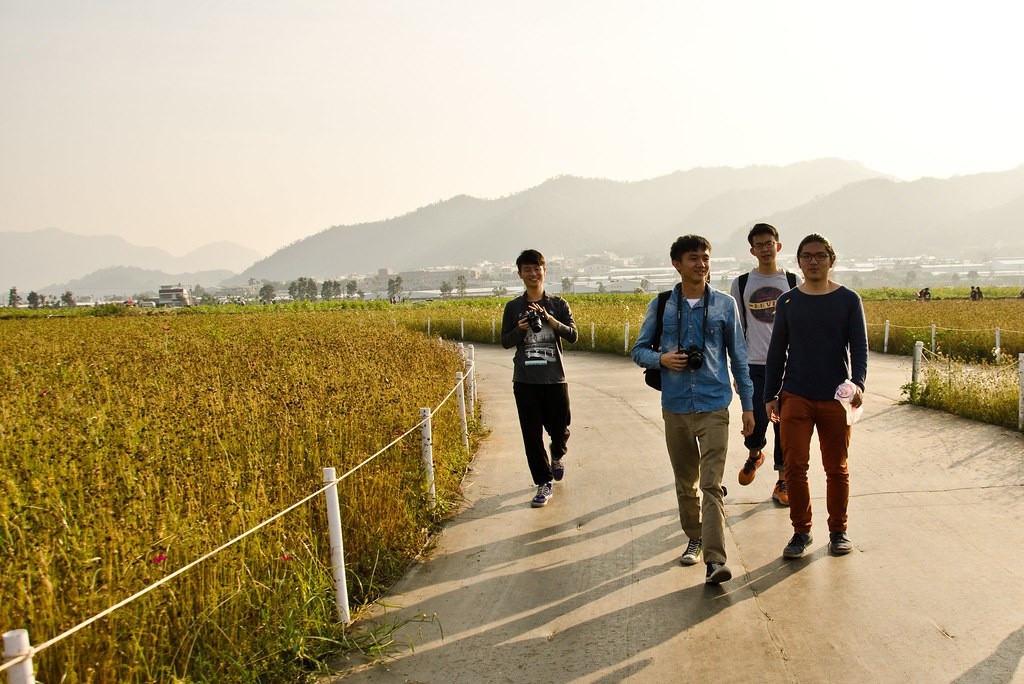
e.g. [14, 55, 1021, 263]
[799, 252, 832, 262]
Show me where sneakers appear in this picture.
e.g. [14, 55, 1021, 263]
[705, 561, 733, 583]
[828, 531, 854, 554]
[551, 459, 565, 481]
[681, 536, 703, 564]
[773, 479, 790, 506]
[783, 532, 814, 558]
[531, 481, 554, 508]
[739, 450, 765, 485]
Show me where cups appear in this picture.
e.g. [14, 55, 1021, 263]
[835, 382, 861, 415]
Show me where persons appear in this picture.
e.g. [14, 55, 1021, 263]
[918, 288, 930, 302]
[970, 286, 983, 301]
[502, 250, 578, 508]
[631, 235, 755, 582]
[393, 297, 397, 304]
[763, 234, 868, 559]
[731, 223, 806, 505]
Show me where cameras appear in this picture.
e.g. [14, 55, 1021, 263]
[522, 309, 545, 333]
[678, 344, 703, 370]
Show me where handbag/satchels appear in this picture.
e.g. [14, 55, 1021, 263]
[643, 290, 672, 390]
